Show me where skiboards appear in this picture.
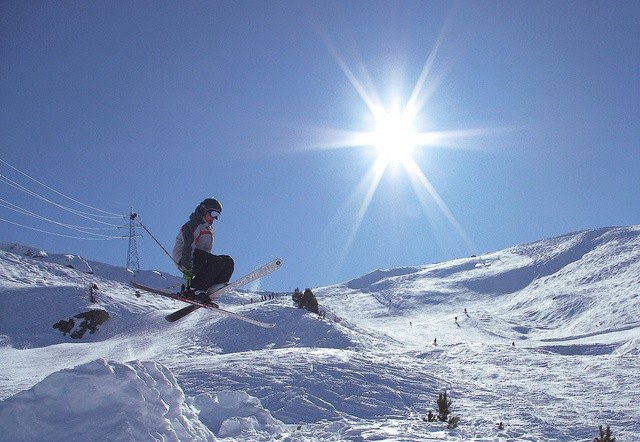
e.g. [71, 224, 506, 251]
[129, 258, 284, 329]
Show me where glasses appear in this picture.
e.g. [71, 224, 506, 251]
[209, 211, 220, 220]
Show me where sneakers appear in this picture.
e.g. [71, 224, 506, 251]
[185, 289, 219, 308]
[208, 282, 230, 295]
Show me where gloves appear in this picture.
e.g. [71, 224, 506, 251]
[183, 268, 192, 286]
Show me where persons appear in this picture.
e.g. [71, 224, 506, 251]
[172, 197, 235, 306]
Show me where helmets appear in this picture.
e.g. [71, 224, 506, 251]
[200, 198, 222, 215]
